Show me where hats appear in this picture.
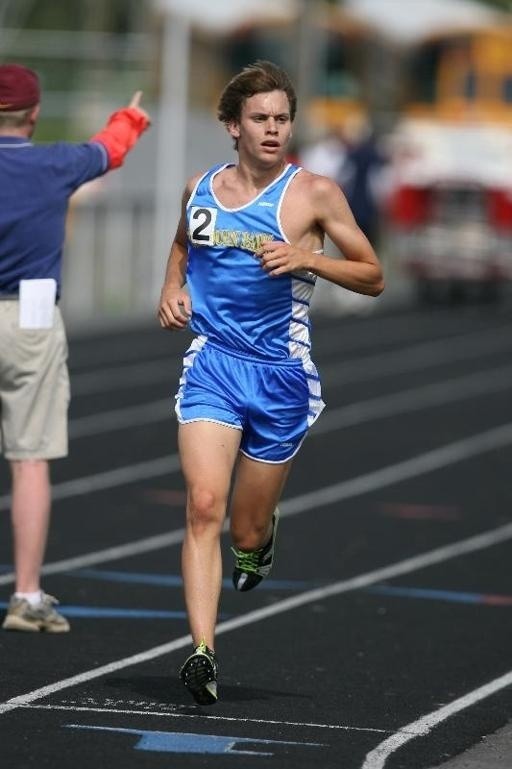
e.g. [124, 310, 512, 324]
[0, 64, 40, 111]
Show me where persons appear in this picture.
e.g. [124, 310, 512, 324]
[157, 59, 385, 705]
[0, 62, 151, 633]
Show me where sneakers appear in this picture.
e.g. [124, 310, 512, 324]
[231, 505, 280, 592]
[178, 647, 218, 705]
[1, 590, 71, 634]
